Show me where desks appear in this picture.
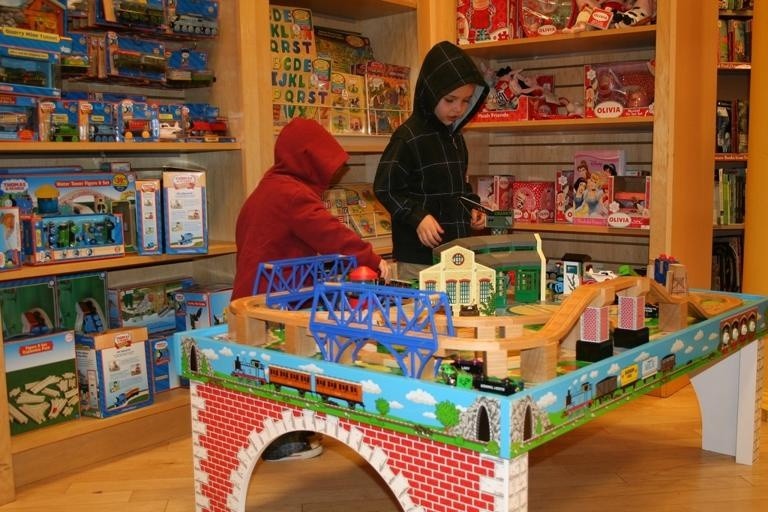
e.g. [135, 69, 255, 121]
[163, 287, 768, 512]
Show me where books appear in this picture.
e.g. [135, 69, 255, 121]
[711, 1, 754, 294]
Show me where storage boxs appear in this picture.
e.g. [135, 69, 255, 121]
[0, 269, 235, 436]
[1, 161, 208, 270]
[509, 180, 556, 225]
[0, 1, 239, 144]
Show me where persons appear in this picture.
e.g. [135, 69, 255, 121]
[370, 41, 491, 283]
[227, 115, 394, 462]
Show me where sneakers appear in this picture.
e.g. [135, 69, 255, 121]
[262, 441, 323, 462]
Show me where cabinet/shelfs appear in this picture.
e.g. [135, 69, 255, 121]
[662, 0, 768, 402]
[187, 2, 449, 258]
[450, 1, 670, 398]
[0, 1, 257, 486]
